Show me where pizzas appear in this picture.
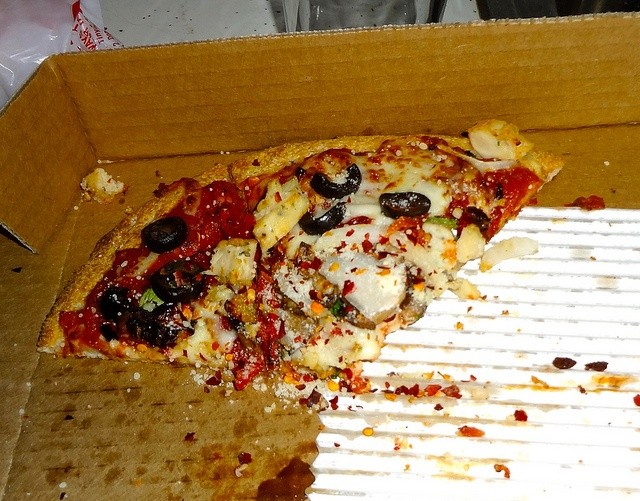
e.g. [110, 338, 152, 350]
[35, 117, 565, 386]
